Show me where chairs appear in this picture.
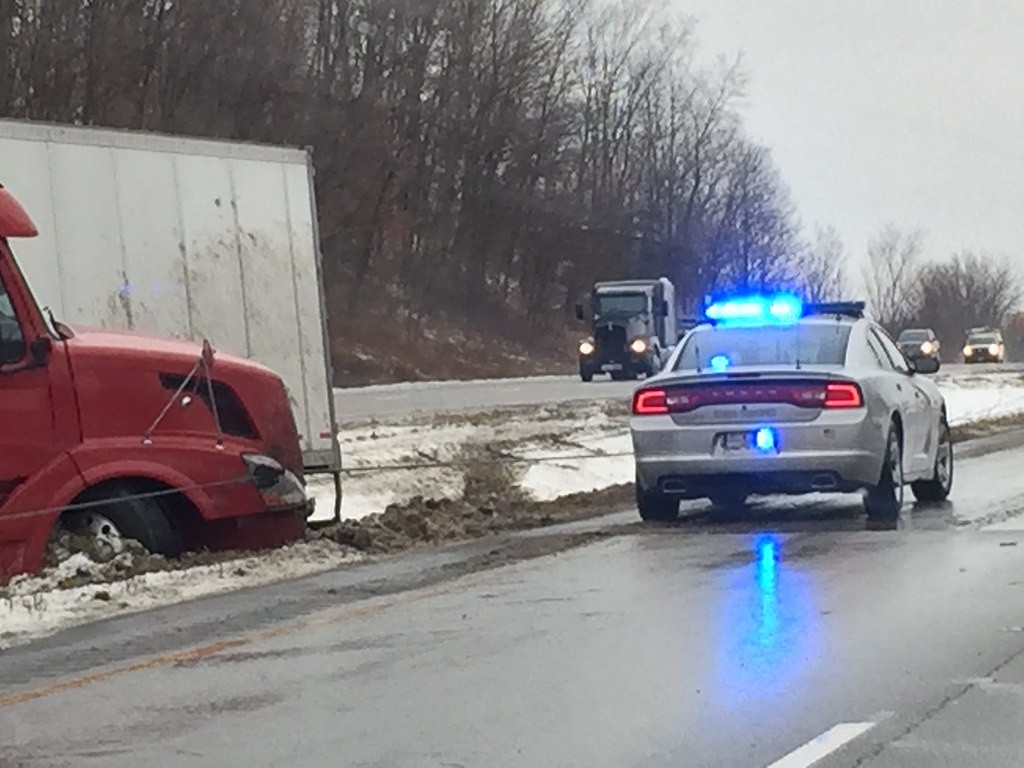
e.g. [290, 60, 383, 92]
[728, 340, 762, 366]
[817, 339, 847, 364]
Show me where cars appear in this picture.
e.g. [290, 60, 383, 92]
[2, 182, 317, 581]
[632, 297, 956, 521]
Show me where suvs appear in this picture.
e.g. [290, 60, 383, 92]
[895, 328, 940, 359]
[964, 327, 1007, 363]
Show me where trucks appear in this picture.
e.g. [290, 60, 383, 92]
[575, 276, 687, 384]
[0, 120, 343, 473]
